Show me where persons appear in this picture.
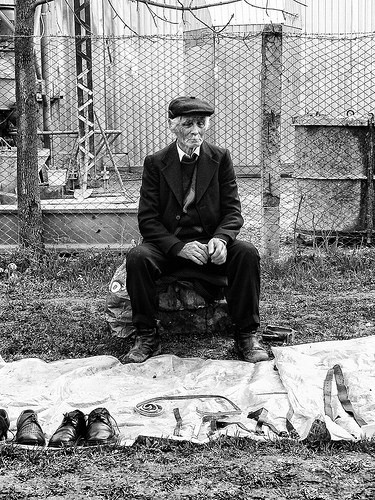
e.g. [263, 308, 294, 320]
[124, 96, 268, 363]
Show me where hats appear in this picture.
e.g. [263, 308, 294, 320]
[168, 96, 214, 119]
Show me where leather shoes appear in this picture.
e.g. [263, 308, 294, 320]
[0, 409, 10, 441]
[48, 410, 84, 447]
[85, 407, 120, 445]
[14, 409, 47, 446]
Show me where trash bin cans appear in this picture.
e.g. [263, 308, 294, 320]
[292, 113, 374, 245]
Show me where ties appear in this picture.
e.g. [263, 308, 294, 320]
[182, 153, 198, 163]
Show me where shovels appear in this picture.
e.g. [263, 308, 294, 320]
[74, 184, 92, 199]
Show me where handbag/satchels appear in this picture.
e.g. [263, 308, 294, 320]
[104, 258, 136, 337]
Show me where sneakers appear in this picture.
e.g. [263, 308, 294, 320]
[234, 334, 270, 362]
[123, 330, 159, 363]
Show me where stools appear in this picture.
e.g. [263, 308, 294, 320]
[163, 278, 229, 334]
